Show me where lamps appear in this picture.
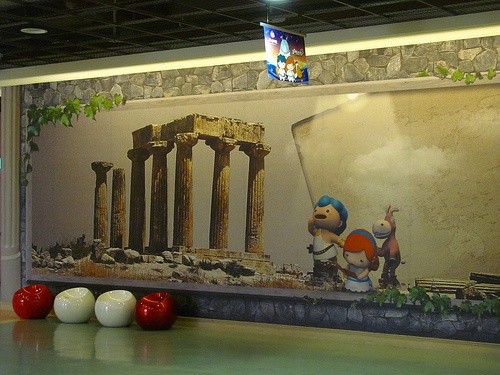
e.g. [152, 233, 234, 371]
[20, 23, 48, 34]
